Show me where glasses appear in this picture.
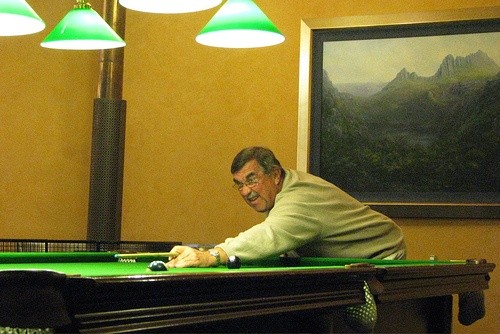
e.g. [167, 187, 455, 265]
[231, 172, 265, 191]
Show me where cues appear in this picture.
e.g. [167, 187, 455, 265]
[115, 252, 179, 258]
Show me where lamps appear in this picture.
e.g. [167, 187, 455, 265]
[0, 0, 286, 251]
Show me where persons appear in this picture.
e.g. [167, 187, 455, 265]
[166, 147, 408, 268]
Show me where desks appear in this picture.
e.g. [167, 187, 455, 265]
[0, 253, 495, 334]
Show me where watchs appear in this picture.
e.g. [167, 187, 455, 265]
[208, 249, 220, 267]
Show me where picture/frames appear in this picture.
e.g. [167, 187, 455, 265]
[297, 7, 500, 219]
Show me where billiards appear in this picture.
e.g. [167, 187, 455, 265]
[226, 256, 241, 269]
[146, 260, 167, 271]
